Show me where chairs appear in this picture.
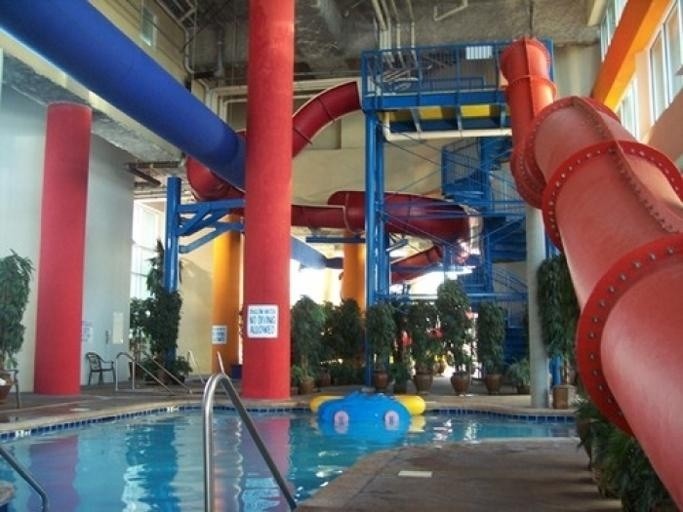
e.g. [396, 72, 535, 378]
[85, 349, 116, 387]
[1, 354, 23, 409]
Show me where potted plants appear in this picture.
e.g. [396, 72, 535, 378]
[126, 289, 193, 386]
[285, 293, 534, 396]
[0, 248, 31, 399]
[535, 256, 581, 410]
